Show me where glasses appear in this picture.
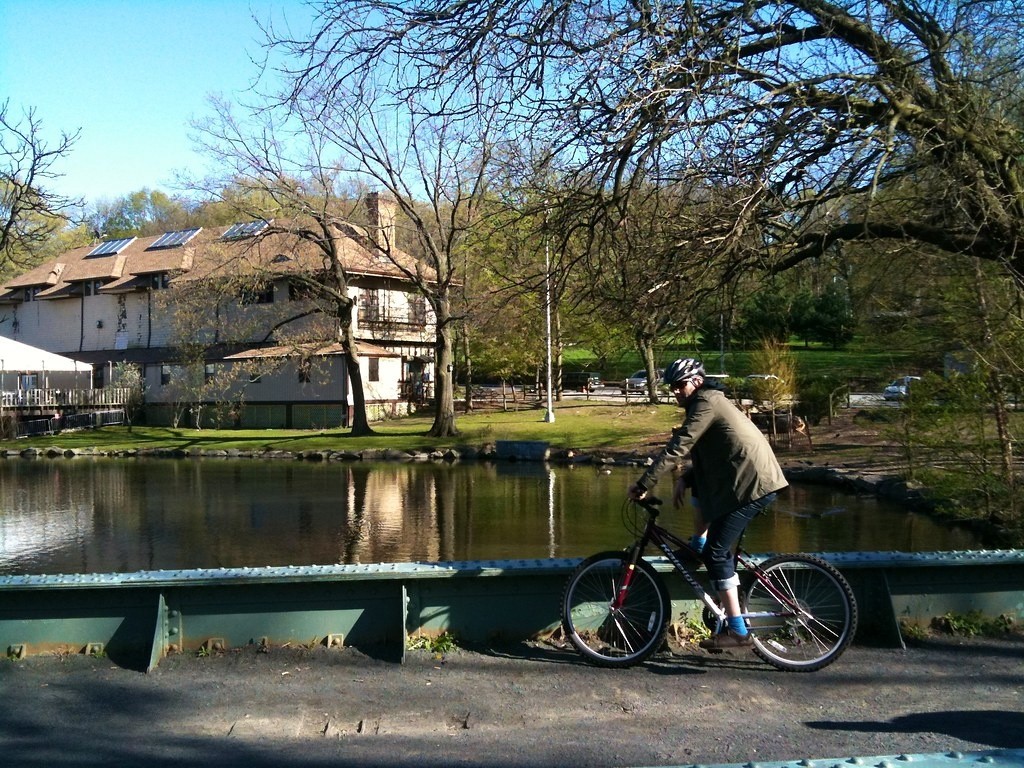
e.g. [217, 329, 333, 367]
[670, 379, 692, 392]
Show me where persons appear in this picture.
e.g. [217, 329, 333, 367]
[628, 359, 789, 649]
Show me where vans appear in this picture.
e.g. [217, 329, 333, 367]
[542, 372, 605, 393]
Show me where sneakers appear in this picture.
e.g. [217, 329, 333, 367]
[672, 543, 702, 570]
[699, 620, 755, 650]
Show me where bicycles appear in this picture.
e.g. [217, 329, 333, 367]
[560, 487, 858, 672]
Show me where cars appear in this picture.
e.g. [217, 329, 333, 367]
[883, 376, 921, 401]
[619, 369, 664, 395]
[747, 374, 785, 383]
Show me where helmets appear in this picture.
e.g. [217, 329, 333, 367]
[664, 358, 705, 388]
